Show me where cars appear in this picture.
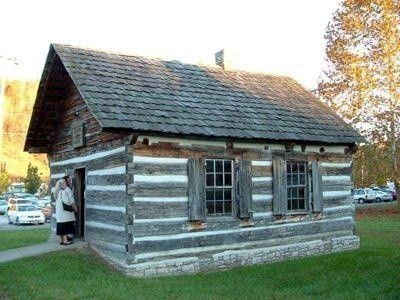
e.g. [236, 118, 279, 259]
[0, 183, 52, 226]
[353, 185, 398, 205]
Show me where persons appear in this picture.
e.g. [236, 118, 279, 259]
[54, 176, 78, 245]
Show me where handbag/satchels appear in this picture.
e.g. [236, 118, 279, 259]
[63, 202, 73, 212]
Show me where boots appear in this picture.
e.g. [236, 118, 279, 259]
[60, 237, 73, 245]
